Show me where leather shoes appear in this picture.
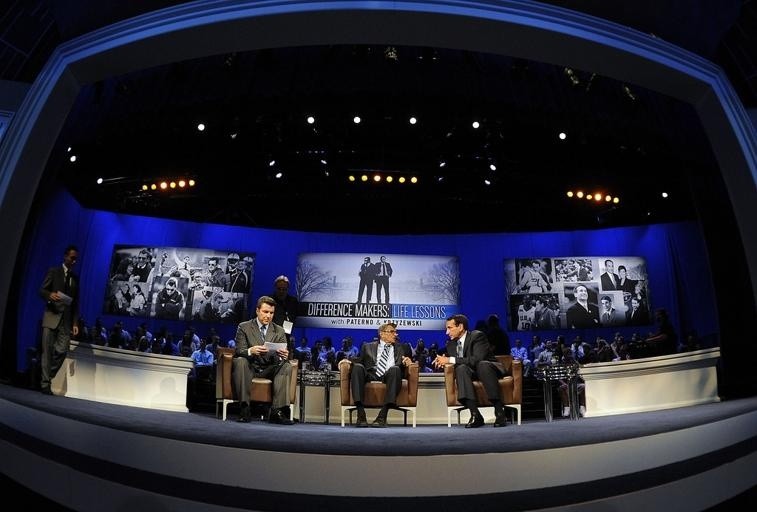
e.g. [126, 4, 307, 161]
[371, 416, 386, 427]
[355, 413, 368, 427]
[236, 409, 249, 422]
[465, 414, 485, 428]
[269, 411, 295, 425]
[494, 412, 508, 427]
[39, 386, 53, 395]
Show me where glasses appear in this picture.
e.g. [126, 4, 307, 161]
[208, 264, 215, 266]
[138, 257, 145, 260]
[167, 288, 174, 292]
[276, 287, 286, 291]
[216, 300, 220, 304]
[383, 330, 397, 334]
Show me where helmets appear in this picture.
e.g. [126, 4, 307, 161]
[227, 253, 239, 267]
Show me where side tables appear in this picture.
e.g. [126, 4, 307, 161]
[535, 363, 580, 423]
[296, 369, 341, 424]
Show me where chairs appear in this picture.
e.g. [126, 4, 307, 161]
[444, 356, 522, 429]
[216, 348, 299, 422]
[340, 362, 419, 427]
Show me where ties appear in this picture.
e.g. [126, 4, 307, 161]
[65, 271, 71, 286]
[457, 342, 463, 358]
[261, 326, 266, 341]
[382, 263, 385, 277]
[375, 344, 391, 377]
[365, 263, 367, 271]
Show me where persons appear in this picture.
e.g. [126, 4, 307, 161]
[76, 306, 681, 381]
[190, 336, 214, 404]
[38, 246, 80, 396]
[432, 314, 508, 428]
[270, 276, 299, 348]
[25, 318, 43, 390]
[176, 345, 198, 411]
[516, 258, 647, 331]
[355, 257, 376, 303]
[557, 356, 588, 421]
[228, 296, 296, 426]
[348, 323, 412, 427]
[110, 248, 253, 317]
[373, 256, 393, 304]
